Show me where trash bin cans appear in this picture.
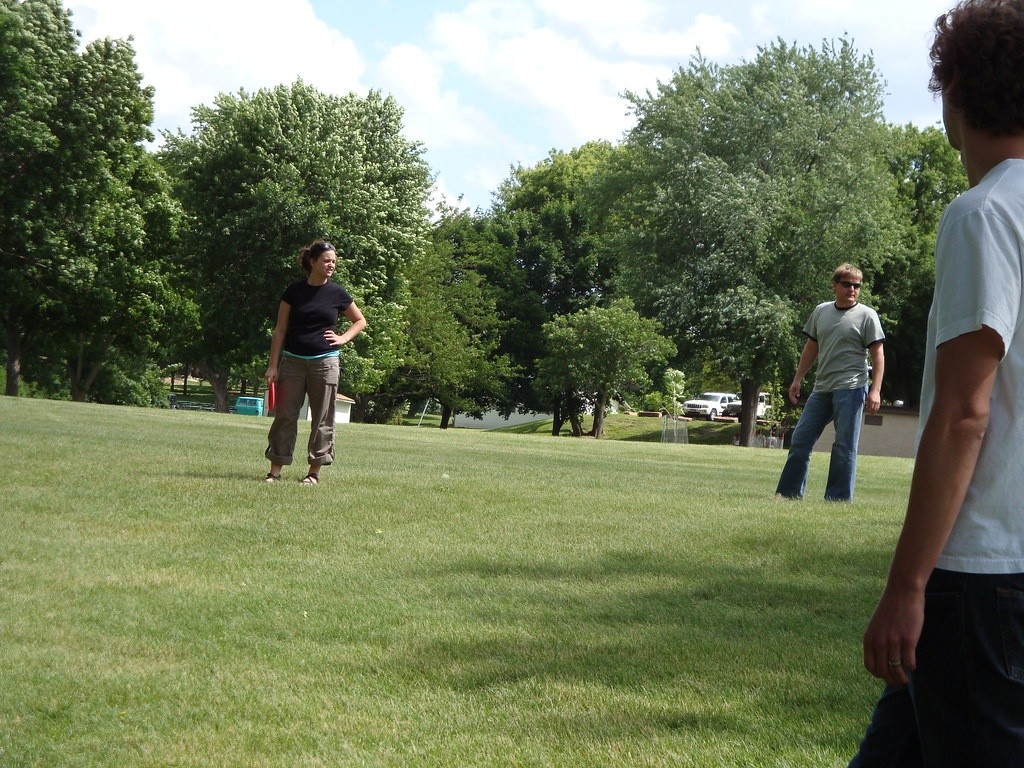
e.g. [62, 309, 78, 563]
[167, 393, 181, 410]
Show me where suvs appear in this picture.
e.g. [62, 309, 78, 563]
[681, 391, 740, 421]
[722, 391, 772, 419]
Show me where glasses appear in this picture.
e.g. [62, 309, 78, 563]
[319, 243, 335, 249]
[839, 280, 862, 288]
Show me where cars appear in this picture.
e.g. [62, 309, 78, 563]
[234, 397, 264, 417]
[166, 393, 234, 412]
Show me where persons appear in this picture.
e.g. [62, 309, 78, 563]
[848, 1, 1023, 768]
[265, 240, 367, 485]
[775, 262, 885, 504]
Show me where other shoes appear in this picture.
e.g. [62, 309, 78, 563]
[265, 473, 281, 482]
[303, 473, 318, 484]
